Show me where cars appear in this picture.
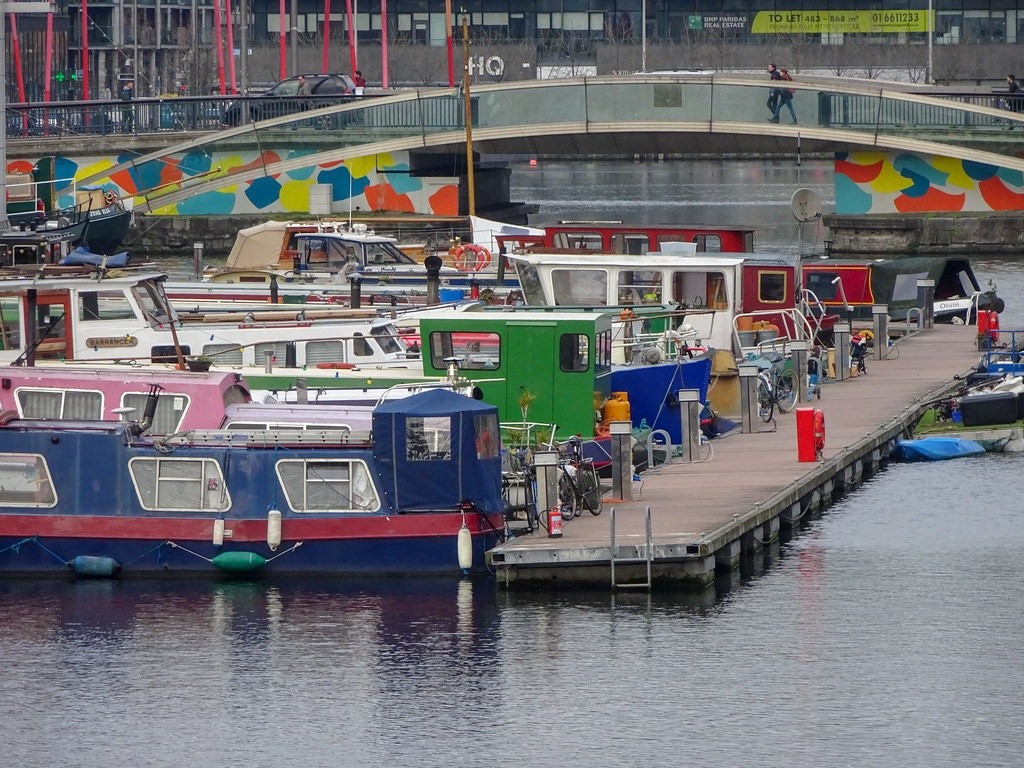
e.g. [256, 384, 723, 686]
[67, 101, 221, 133]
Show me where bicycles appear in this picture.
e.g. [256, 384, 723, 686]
[753, 354, 801, 423]
[541, 435, 603, 521]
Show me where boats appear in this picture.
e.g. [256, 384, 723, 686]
[0, 176, 968, 575]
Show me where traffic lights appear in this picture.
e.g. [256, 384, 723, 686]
[51, 71, 78, 84]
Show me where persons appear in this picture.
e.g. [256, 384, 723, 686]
[1006, 75, 1024, 131]
[766, 62, 798, 125]
[291, 75, 321, 131]
[121, 82, 134, 135]
[353, 71, 367, 126]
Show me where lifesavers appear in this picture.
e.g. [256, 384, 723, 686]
[315, 361, 357, 371]
[475, 246, 493, 268]
[450, 243, 486, 272]
[654, 329, 685, 364]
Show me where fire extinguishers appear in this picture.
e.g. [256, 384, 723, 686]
[537, 504, 565, 539]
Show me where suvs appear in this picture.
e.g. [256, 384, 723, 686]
[224, 73, 364, 130]
